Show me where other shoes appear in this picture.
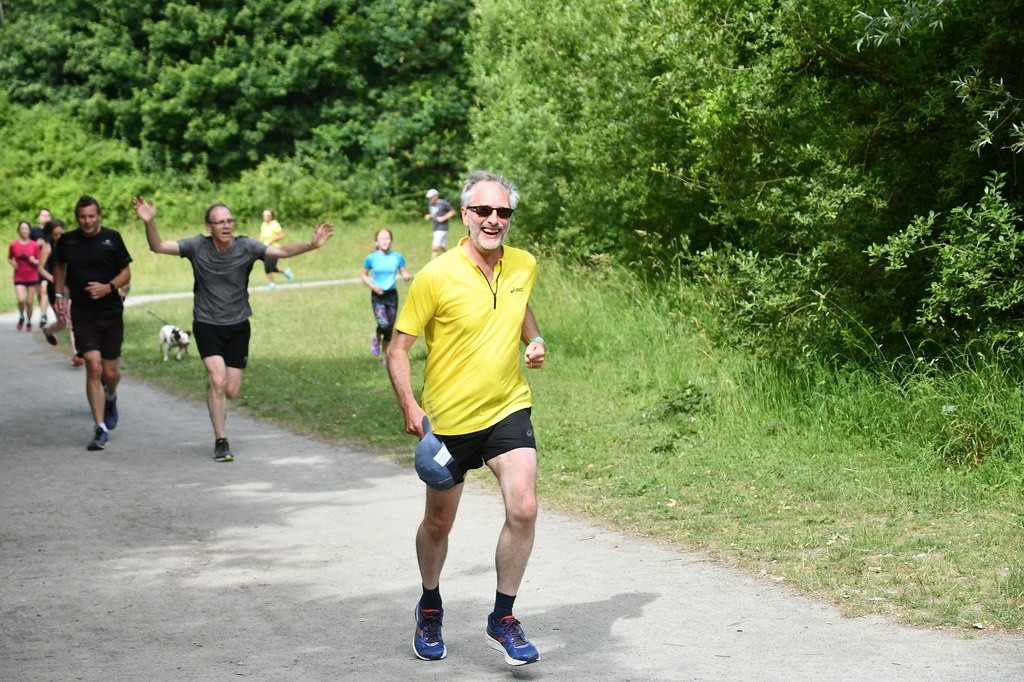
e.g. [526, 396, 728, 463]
[268, 283, 275, 289]
[284, 267, 292, 281]
[71, 354, 84, 366]
[17, 317, 25, 330]
[43, 325, 57, 345]
[26, 322, 31, 331]
[371, 336, 380, 356]
[40, 315, 47, 327]
[381, 357, 387, 365]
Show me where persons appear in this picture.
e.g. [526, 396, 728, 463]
[260, 210, 292, 287]
[424, 189, 456, 260]
[8, 208, 84, 365]
[53, 195, 133, 450]
[386, 171, 546, 666]
[132, 196, 335, 461]
[361, 227, 412, 365]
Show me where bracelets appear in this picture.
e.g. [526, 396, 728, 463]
[55, 292, 65, 299]
[109, 282, 115, 293]
[531, 337, 545, 343]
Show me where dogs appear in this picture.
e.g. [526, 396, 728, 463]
[158, 325, 192, 362]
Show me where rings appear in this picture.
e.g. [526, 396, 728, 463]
[530, 363, 532, 367]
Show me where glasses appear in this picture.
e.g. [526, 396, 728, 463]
[208, 218, 236, 225]
[466, 206, 514, 219]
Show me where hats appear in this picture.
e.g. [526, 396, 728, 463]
[426, 189, 439, 198]
[414, 416, 456, 491]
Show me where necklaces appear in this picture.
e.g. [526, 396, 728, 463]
[477, 260, 502, 309]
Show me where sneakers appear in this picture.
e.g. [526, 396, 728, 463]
[87, 425, 108, 450]
[214, 438, 235, 462]
[412, 601, 447, 660]
[104, 387, 118, 430]
[485, 611, 540, 666]
